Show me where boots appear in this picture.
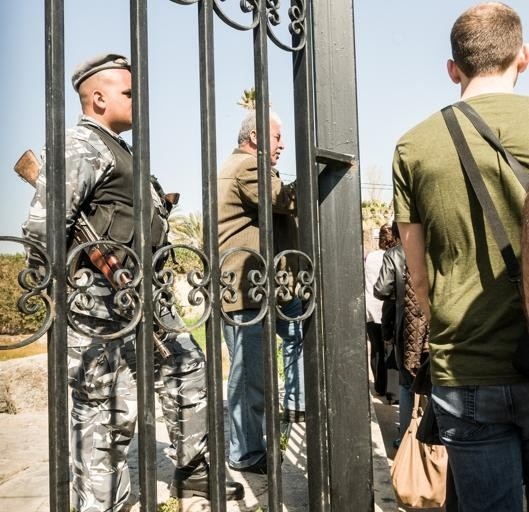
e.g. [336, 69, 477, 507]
[171, 458, 244, 500]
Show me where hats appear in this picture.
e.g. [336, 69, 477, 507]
[71, 52, 131, 92]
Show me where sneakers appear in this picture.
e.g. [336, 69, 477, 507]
[229, 453, 283, 474]
[284, 408, 304, 423]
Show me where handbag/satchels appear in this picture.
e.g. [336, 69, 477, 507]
[390, 415, 449, 510]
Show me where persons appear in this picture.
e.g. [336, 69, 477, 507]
[391, 0, 528, 512]
[21, 52, 247, 512]
[363, 222, 433, 450]
[214, 110, 300, 477]
[272, 182, 307, 425]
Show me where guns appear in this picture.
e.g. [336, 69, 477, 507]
[13, 148, 172, 363]
